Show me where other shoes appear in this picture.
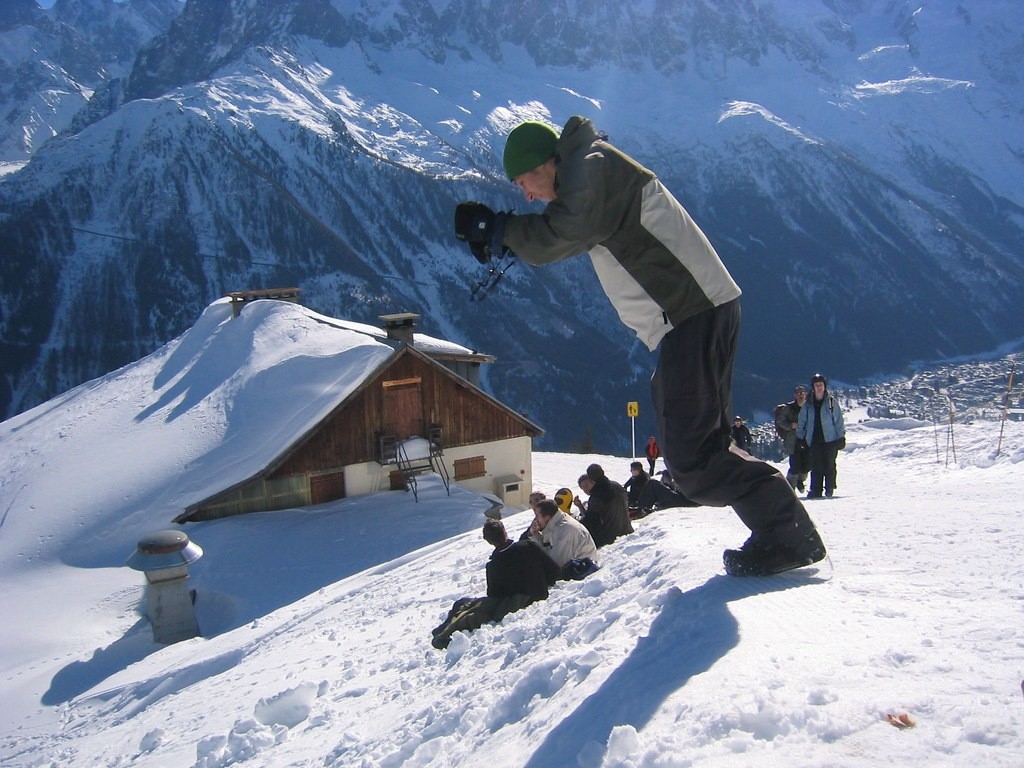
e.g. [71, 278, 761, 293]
[797, 481, 804, 493]
[806, 489, 823, 499]
[628, 510, 642, 519]
[825, 489, 833, 497]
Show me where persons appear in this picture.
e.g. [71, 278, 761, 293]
[431, 375, 846, 650]
[454, 115, 825, 578]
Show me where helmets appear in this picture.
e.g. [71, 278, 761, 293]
[794, 386, 806, 394]
[561, 556, 600, 581]
[811, 373, 826, 389]
[734, 416, 742, 424]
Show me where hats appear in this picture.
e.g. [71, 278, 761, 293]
[503, 121, 560, 180]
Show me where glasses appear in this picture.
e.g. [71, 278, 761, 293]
[734, 421, 741, 425]
[797, 391, 806, 396]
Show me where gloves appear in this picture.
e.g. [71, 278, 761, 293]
[469, 242, 493, 264]
[838, 437, 845, 450]
[454, 201, 516, 259]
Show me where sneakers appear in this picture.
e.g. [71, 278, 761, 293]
[723, 524, 826, 578]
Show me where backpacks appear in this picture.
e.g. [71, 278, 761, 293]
[773, 402, 799, 439]
